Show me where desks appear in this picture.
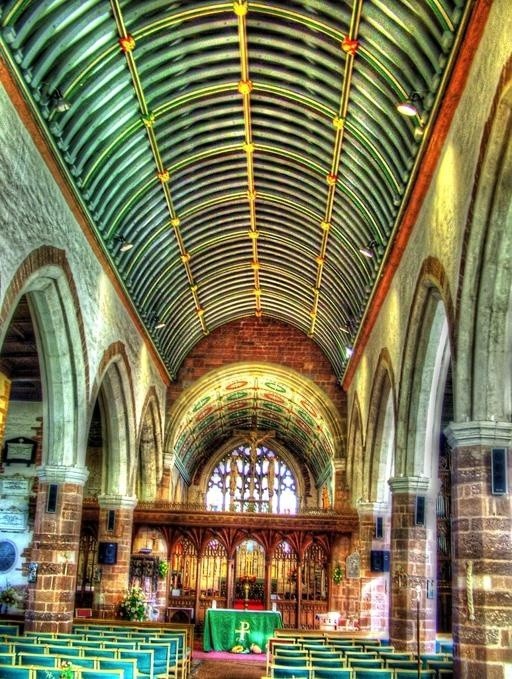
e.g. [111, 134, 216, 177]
[205, 607, 281, 654]
[166, 606, 194, 624]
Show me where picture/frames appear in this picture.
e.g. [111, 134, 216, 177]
[4, 434, 38, 467]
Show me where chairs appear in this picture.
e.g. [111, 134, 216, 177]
[258, 627, 452, 679]
[0, 617, 196, 679]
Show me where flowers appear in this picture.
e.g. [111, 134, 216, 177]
[119, 585, 149, 621]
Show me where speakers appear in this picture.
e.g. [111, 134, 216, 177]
[375, 517, 384, 539]
[414, 495, 426, 526]
[98, 541, 118, 565]
[107, 509, 115, 532]
[371, 550, 390, 572]
[490, 447, 509, 495]
[46, 483, 58, 513]
